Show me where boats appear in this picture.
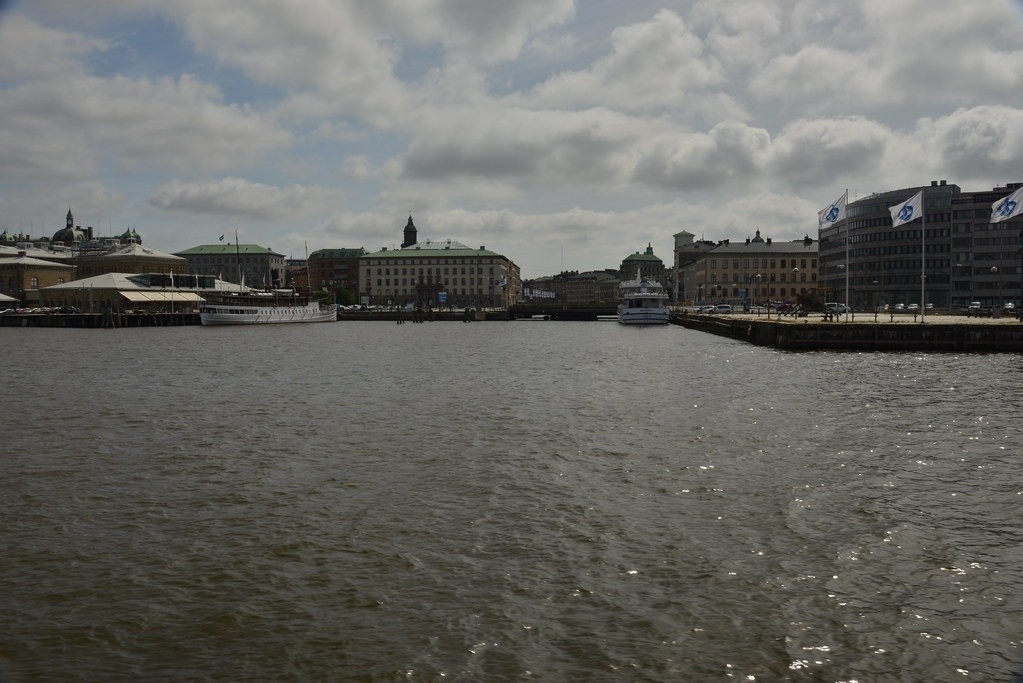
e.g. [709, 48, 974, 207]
[200, 289, 336, 326]
[616, 267, 670, 324]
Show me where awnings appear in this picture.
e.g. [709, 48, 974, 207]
[117, 290, 207, 302]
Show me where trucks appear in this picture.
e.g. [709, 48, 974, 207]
[824, 302, 851, 313]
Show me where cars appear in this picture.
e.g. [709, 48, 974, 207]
[895, 304, 905, 310]
[694, 305, 719, 314]
[715, 304, 732, 314]
[907, 303, 919, 310]
[925, 303, 934, 310]
[0, 305, 80, 315]
[969, 302, 982, 308]
[1002, 303, 1015, 313]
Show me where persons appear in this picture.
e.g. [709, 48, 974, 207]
[92, 298, 96, 312]
[87, 296, 91, 312]
[101, 297, 106, 312]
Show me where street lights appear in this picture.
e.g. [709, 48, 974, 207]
[793, 268, 800, 319]
[990, 266, 997, 319]
[756, 274, 761, 318]
[714, 285, 717, 299]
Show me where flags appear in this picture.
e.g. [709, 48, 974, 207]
[887, 190, 922, 228]
[219, 235, 224, 241]
[818, 194, 846, 230]
[990, 186, 1023, 223]
